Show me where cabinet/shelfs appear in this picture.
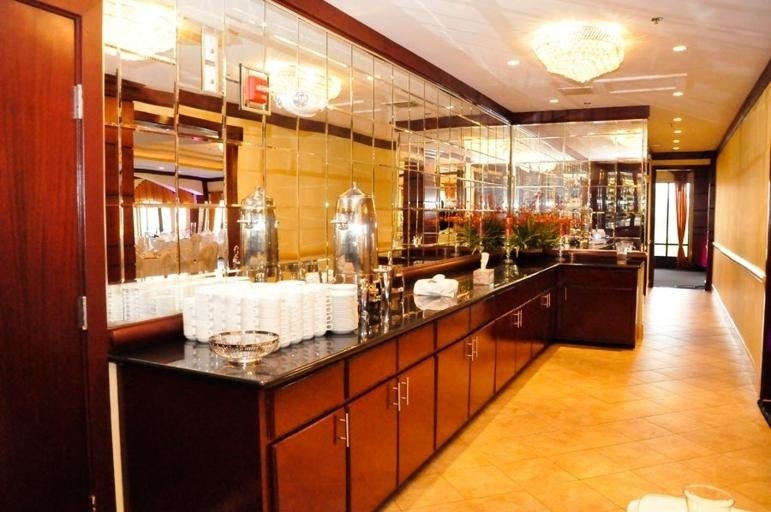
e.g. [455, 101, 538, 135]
[555, 262, 646, 350]
[116, 358, 350, 511]
[345, 266, 561, 512]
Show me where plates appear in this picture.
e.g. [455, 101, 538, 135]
[330, 284, 359, 334]
[182, 280, 333, 353]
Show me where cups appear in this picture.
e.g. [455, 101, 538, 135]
[621, 241, 636, 251]
[615, 241, 631, 260]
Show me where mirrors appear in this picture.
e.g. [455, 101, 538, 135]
[513, 119, 647, 254]
[102, 0, 511, 329]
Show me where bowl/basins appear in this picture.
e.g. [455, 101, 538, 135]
[208, 330, 280, 367]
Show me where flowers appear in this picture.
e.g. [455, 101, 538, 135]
[508, 191, 584, 258]
[437, 194, 508, 256]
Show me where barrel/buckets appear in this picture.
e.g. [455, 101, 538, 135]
[472, 251, 494, 286]
